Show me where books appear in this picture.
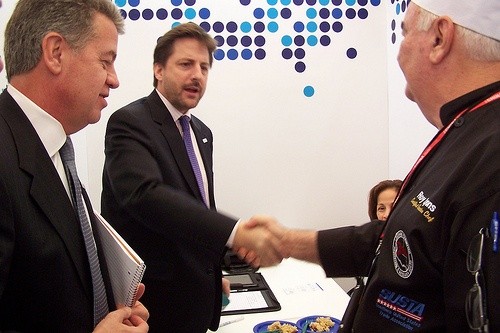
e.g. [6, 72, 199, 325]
[92, 210, 146, 310]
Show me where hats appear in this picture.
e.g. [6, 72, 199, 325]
[410, 0, 500, 41]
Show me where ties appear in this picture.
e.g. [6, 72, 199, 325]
[58, 133, 109, 329]
[176, 115, 207, 205]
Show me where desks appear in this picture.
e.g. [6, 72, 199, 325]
[205, 257, 351, 333]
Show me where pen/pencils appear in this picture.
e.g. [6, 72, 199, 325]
[238, 287, 268, 292]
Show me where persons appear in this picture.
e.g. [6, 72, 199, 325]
[100, 22, 290, 333]
[367, 180, 404, 222]
[233, 0, 500, 333]
[0, 0, 150, 333]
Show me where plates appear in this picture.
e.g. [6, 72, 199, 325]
[252, 320, 298, 333]
[296, 315, 342, 333]
